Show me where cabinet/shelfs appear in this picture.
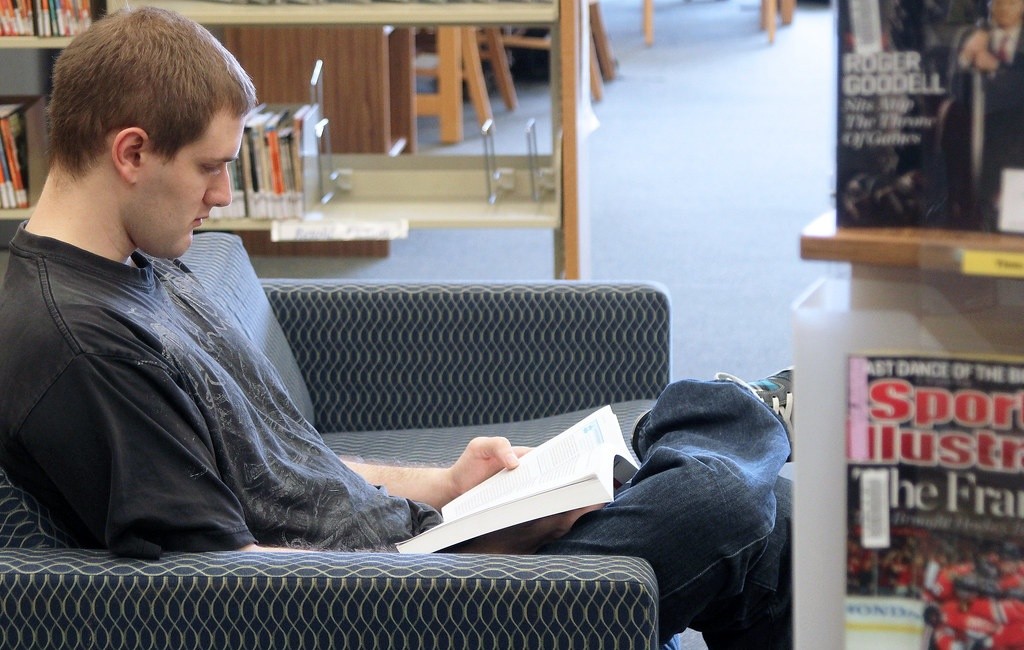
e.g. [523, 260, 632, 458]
[0, 0, 589, 284]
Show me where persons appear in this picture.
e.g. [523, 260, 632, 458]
[0, 8, 793, 650]
[941, 0, 1024, 230]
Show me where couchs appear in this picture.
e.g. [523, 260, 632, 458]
[0, 232, 672, 650]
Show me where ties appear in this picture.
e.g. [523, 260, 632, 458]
[999, 36, 1012, 64]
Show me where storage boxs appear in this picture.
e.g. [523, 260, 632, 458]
[787, 279, 1024, 650]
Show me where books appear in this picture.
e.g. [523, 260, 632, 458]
[395, 404, 639, 556]
[0, 0, 94, 36]
[832, 1, 1024, 235]
[0, 101, 30, 209]
[844, 349, 1024, 650]
[209, 102, 320, 219]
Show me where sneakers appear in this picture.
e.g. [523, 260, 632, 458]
[631, 365, 795, 463]
